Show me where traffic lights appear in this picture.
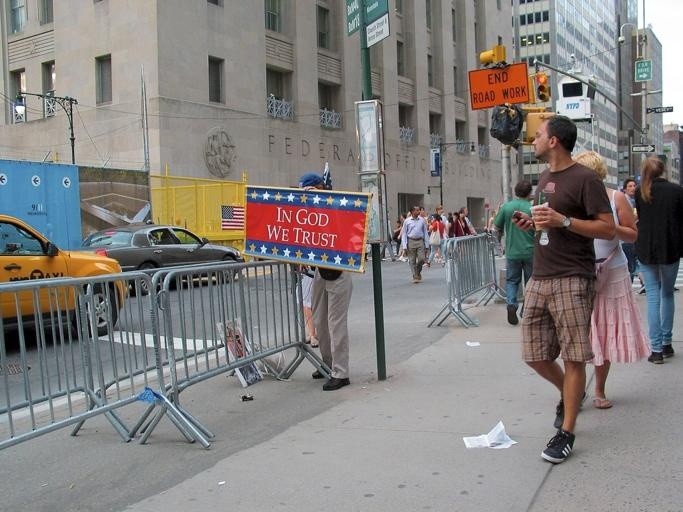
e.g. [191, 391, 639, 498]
[535, 72, 549, 103]
[532, 73, 550, 104]
[479, 44, 505, 66]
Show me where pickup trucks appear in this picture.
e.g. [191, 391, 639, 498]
[1, 215, 129, 337]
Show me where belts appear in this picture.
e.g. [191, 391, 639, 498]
[407, 236, 423, 241]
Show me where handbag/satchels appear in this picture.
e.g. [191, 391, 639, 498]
[594, 247, 620, 291]
[428, 221, 442, 246]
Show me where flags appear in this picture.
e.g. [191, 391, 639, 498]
[221, 204, 245, 230]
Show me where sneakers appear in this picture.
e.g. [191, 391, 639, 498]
[508, 304, 518, 326]
[663, 344, 675, 358]
[554, 388, 589, 429]
[542, 430, 575, 464]
[647, 352, 664, 364]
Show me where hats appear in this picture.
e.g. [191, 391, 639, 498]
[300, 173, 323, 187]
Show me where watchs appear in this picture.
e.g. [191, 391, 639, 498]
[562, 216, 572, 231]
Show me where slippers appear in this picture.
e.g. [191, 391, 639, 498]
[592, 396, 613, 409]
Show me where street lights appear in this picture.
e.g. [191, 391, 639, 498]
[438, 140, 476, 205]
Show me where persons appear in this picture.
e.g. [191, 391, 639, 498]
[289, 172, 354, 391]
[392, 204, 479, 283]
[619, 179, 638, 284]
[296, 267, 320, 349]
[494, 180, 541, 325]
[635, 157, 683, 364]
[487, 210, 498, 251]
[511, 115, 617, 464]
[571, 150, 650, 409]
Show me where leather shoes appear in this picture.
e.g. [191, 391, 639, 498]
[323, 376, 350, 391]
[313, 365, 327, 379]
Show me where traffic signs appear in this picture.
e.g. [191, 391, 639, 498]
[646, 106, 673, 114]
[367, 12, 390, 48]
[345, 0, 390, 47]
[466, 63, 530, 111]
[631, 144, 656, 153]
[635, 60, 653, 82]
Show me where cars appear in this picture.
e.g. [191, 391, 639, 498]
[80, 223, 242, 294]
